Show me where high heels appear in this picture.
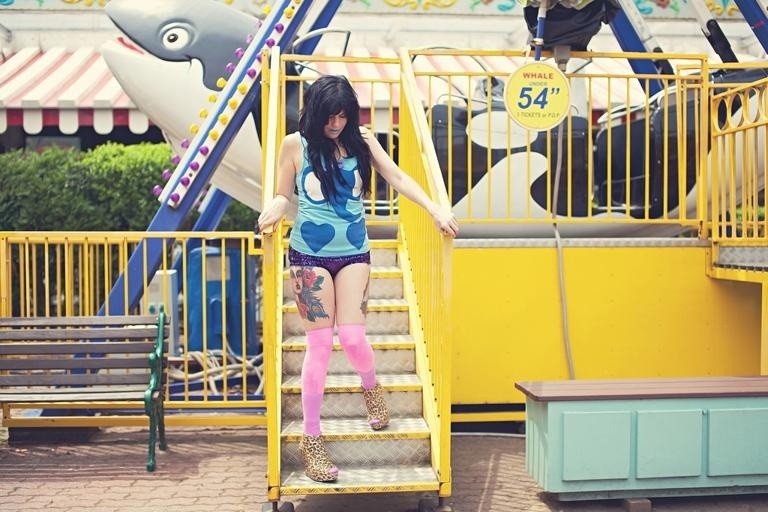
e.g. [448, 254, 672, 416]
[297, 431, 339, 482]
[358, 380, 391, 431]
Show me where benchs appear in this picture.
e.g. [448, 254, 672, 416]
[0, 312, 168, 475]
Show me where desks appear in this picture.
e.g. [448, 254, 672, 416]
[514, 375, 768, 503]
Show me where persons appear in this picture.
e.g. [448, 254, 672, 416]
[257, 73, 460, 482]
